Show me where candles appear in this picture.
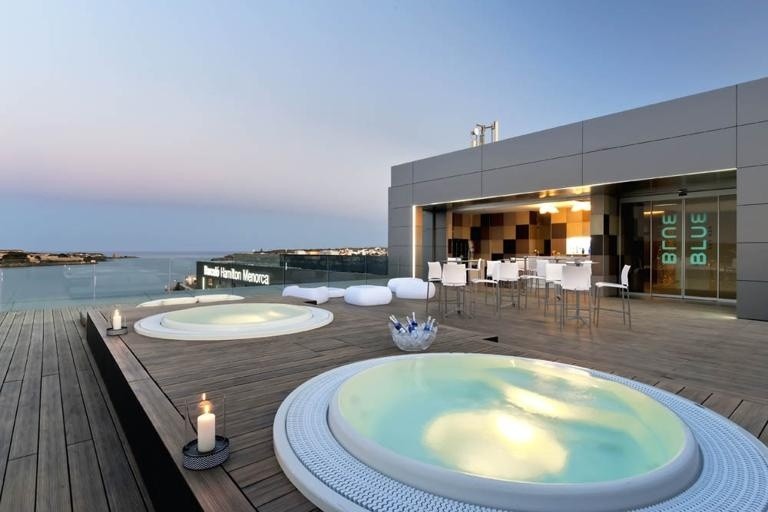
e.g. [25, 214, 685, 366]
[197, 409, 217, 453]
[111, 309, 122, 330]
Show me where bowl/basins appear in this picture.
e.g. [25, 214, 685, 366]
[388, 322, 439, 352]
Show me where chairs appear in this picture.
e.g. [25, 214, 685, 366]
[427, 250, 634, 333]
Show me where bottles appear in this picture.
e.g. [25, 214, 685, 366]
[389, 311, 437, 341]
